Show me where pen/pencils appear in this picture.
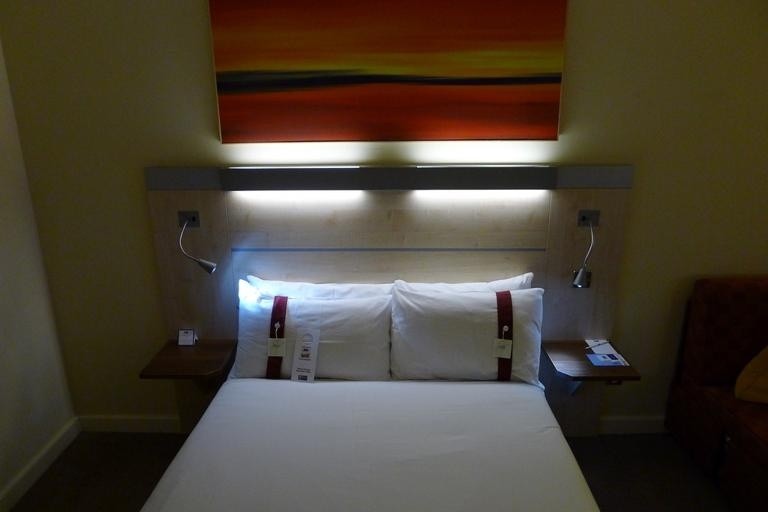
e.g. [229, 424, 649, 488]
[584, 340, 610, 349]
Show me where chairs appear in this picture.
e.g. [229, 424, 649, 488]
[661, 271, 768, 511]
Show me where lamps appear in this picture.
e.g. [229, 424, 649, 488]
[171, 212, 220, 274]
[571, 209, 602, 291]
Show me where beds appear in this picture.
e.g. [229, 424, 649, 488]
[133, 379, 605, 512]
